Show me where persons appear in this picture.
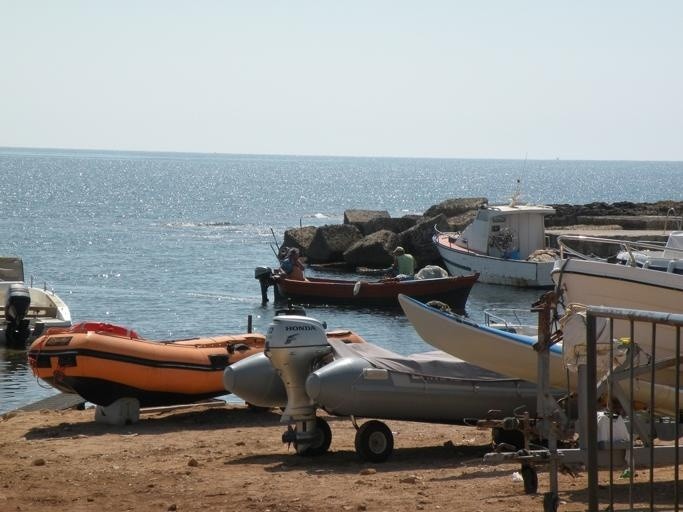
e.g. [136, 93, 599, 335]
[390, 246, 417, 279]
[277, 247, 304, 279]
[595, 398, 634, 471]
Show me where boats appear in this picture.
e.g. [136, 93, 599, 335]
[254, 266, 479, 308]
[222, 314, 566, 427]
[432, 149, 608, 290]
[26, 320, 365, 410]
[550, 229, 682, 412]
[0, 257, 72, 349]
[398, 294, 683, 417]
